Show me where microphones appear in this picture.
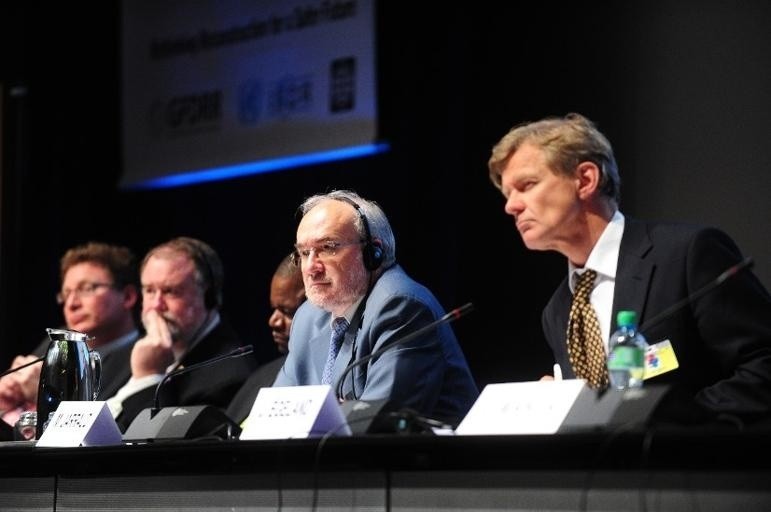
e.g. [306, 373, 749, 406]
[121, 344, 255, 441]
[335, 301, 475, 435]
[0, 356, 47, 441]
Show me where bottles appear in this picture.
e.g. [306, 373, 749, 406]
[601, 308, 650, 391]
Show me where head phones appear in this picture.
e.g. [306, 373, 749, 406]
[289, 196, 384, 274]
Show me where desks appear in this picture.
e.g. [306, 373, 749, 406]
[1, 431, 771, 512]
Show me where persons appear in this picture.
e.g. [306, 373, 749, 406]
[482, 109, 770, 419]
[1, 239, 143, 425]
[98, 232, 256, 432]
[271, 188, 481, 430]
[224, 252, 310, 424]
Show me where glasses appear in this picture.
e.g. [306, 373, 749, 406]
[56, 281, 111, 304]
[296, 240, 363, 258]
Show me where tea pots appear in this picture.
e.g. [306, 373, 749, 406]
[38, 325, 105, 441]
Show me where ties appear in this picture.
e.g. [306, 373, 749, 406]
[321, 316, 349, 388]
[566, 269, 610, 393]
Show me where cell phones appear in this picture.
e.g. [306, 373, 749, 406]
[328, 58, 356, 113]
